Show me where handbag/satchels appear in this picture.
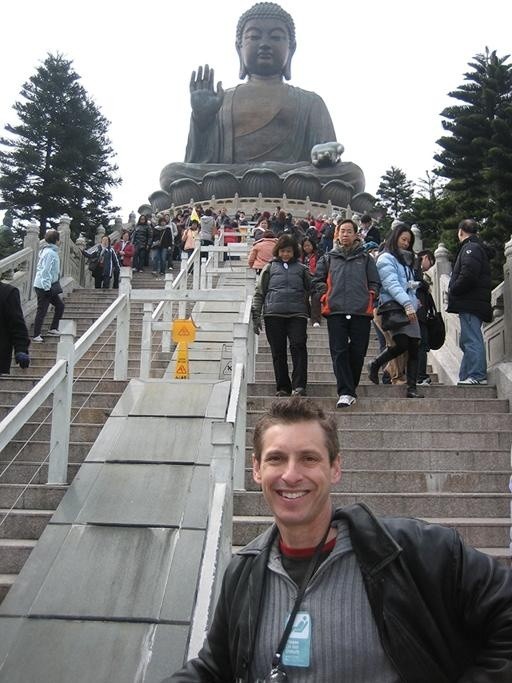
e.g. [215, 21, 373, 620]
[428, 312, 446, 350]
[88, 258, 99, 270]
[376, 301, 410, 331]
[151, 240, 159, 249]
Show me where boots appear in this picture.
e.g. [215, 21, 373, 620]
[367, 347, 396, 384]
[406, 358, 424, 398]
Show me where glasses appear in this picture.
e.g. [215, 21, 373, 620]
[429, 257, 433, 267]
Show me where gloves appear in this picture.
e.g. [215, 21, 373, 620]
[253, 317, 262, 335]
[15, 351, 30, 369]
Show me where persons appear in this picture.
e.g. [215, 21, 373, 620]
[33, 230, 64, 341]
[248, 230, 279, 283]
[250, 205, 344, 234]
[133, 203, 247, 276]
[356, 214, 381, 245]
[413, 250, 436, 386]
[297, 236, 325, 328]
[252, 235, 326, 397]
[0, 280, 31, 374]
[160, 397, 512, 683]
[81, 236, 120, 289]
[160, 1, 366, 191]
[366, 223, 423, 397]
[112, 231, 136, 288]
[445, 219, 493, 386]
[313, 218, 381, 406]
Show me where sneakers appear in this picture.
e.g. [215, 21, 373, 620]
[275, 391, 289, 397]
[31, 335, 46, 344]
[381, 371, 393, 384]
[457, 377, 488, 385]
[169, 267, 173, 270]
[139, 270, 143, 273]
[151, 271, 156, 274]
[416, 378, 432, 386]
[161, 273, 164, 275]
[313, 322, 320, 327]
[46, 330, 60, 337]
[336, 394, 356, 407]
[291, 387, 306, 397]
